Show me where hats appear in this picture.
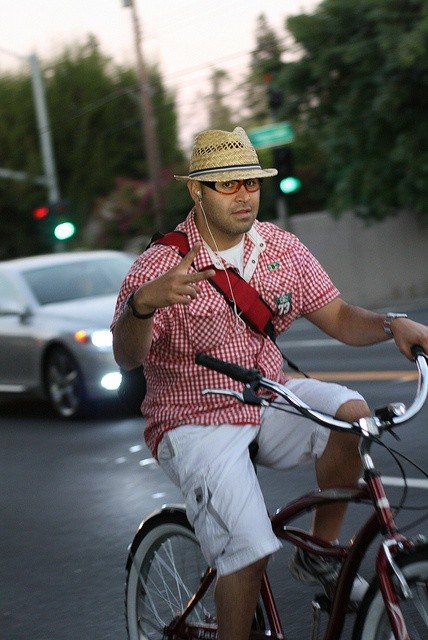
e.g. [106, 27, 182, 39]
[174, 126, 279, 183]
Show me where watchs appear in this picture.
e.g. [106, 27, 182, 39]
[384, 311, 408, 338]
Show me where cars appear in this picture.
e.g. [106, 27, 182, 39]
[0, 250, 137, 419]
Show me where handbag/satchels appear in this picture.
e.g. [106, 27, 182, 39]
[117, 370, 142, 417]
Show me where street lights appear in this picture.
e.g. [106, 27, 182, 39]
[1, 47, 61, 204]
[121, 1, 166, 228]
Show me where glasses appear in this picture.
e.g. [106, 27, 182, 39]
[200, 177, 261, 195]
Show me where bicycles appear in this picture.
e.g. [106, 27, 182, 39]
[124, 344, 427, 639]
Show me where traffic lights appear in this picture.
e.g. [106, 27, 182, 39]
[278, 177, 301, 194]
[52, 218, 76, 240]
[31, 204, 51, 219]
[61, 156, 92, 192]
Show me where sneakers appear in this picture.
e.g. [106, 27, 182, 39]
[288, 546, 370, 613]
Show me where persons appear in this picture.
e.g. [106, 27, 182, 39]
[111, 129, 427, 637]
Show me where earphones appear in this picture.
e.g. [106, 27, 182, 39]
[195, 190, 201, 201]
